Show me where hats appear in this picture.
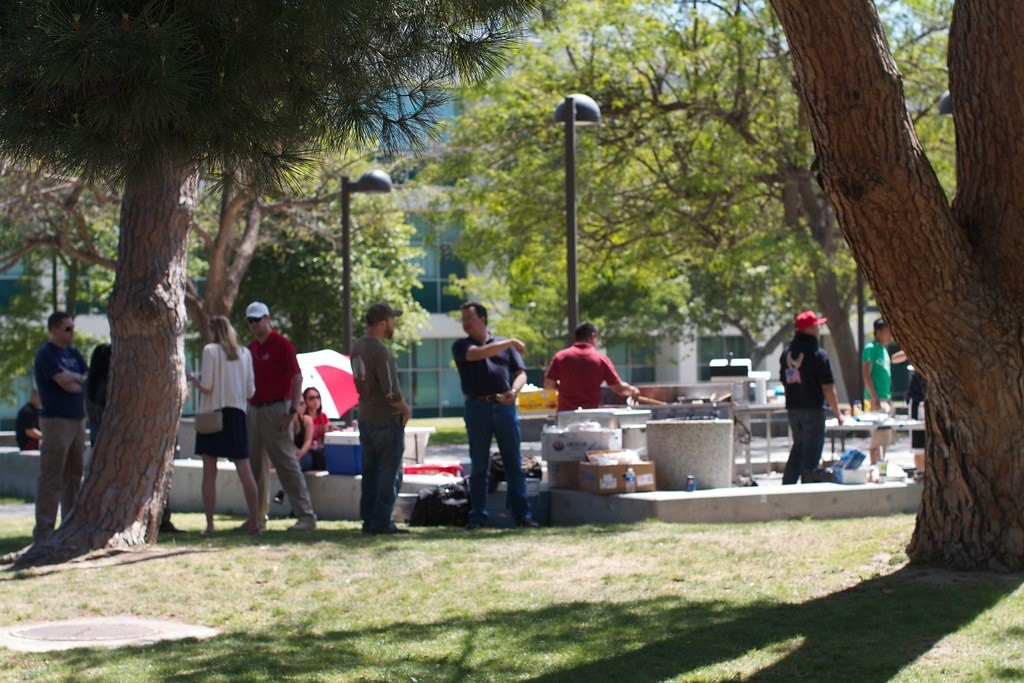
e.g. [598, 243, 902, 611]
[245, 301, 270, 318]
[367, 304, 403, 323]
[796, 311, 828, 331]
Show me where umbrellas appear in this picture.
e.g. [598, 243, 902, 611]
[296, 349, 360, 419]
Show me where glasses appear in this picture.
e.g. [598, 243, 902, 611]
[300, 401, 305, 406]
[58, 326, 75, 332]
[308, 396, 320, 400]
[247, 315, 266, 324]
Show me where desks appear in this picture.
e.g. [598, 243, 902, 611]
[595, 402, 787, 490]
[827, 423, 925, 482]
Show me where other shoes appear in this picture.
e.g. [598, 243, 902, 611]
[233, 525, 263, 536]
[515, 515, 540, 528]
[286, 510, 295, 518]
[200, 530, 214, 536]
[464, 517, 481, 532]
[159, 520, 187, 533]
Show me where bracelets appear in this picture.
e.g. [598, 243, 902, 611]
[510, 388, 517, 394]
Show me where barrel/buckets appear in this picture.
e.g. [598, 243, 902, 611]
[498, 479, 539, 523]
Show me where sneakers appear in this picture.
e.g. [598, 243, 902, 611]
[242, 521, 267, 529]
[287, 521, 318, 531]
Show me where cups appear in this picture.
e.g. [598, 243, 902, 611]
[877, 461, 887, 483]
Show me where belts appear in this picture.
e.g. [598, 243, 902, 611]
[250, 396, 286, 407]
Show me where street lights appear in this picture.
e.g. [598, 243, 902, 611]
[341, 168, 392, 355]
[855, 88, 957, 411]
[552, 90, 604, 346]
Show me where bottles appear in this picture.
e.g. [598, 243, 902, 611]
[625, 468, 636, 493]
[918, 402, 925, 419]
[853, 400, 863, 422]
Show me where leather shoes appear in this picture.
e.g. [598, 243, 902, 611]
[361, 523, 410, 533]
[272, 489, 285, 504]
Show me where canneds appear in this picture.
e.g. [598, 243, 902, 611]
[686, 476, 695, 491]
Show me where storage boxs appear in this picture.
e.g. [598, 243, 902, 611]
[834, 468, 867, 484]
[540, 431, 622, 463]
[516, 388, 559, 413]
[546, 461, 580, 490]
[579, 449, 656, 494]
[404, 426, 435, 465]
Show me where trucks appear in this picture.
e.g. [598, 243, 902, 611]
[710, 357, 772, 403]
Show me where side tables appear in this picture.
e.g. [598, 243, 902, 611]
[324, 429, 363, 476]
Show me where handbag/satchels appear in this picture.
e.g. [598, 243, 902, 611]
[410, 483, 470, 527]
[488, 451, 542, 492]
[195, 412, 223, 434]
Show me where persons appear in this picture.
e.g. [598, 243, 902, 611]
[273, 387, 343, 504]
[861, 317, 926, 473]
[245, 300, 318, 533]
[186, 316, 261, 538]
[350, 302, 412, 536]
[779, 311, 844, 485]
[543, 322, 641, 426]
[15, 384, 44, 452]
[31, 310, 90, 544]
[451, 300, 541, 530]
